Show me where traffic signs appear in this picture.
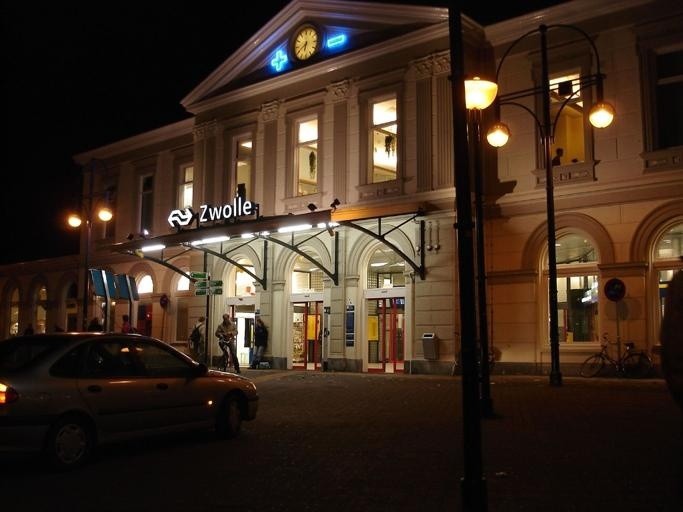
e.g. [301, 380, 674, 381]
[190, 267, 224, 365]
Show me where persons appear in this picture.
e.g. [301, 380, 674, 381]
[187, 316, 206, 354]
[119, 314, 131, 333]
[54, 322, 65, 332]
[23, 323, 35, 335]
[247, 318, 268, 369]
[215, 314, 241, 373]
[88, 317, 104, 330]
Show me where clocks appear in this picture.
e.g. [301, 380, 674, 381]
[286, 22, 326, 68]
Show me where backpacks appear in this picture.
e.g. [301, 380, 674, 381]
[190, 324, 203, 342]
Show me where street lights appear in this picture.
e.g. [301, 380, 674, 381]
[61, 156, 118, 330]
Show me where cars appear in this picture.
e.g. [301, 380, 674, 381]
[1, 328, 262, 469]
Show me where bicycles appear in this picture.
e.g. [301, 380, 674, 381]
[576, 330, 654, 379]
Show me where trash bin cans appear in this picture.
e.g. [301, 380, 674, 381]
[422, 333, 439, 360]
